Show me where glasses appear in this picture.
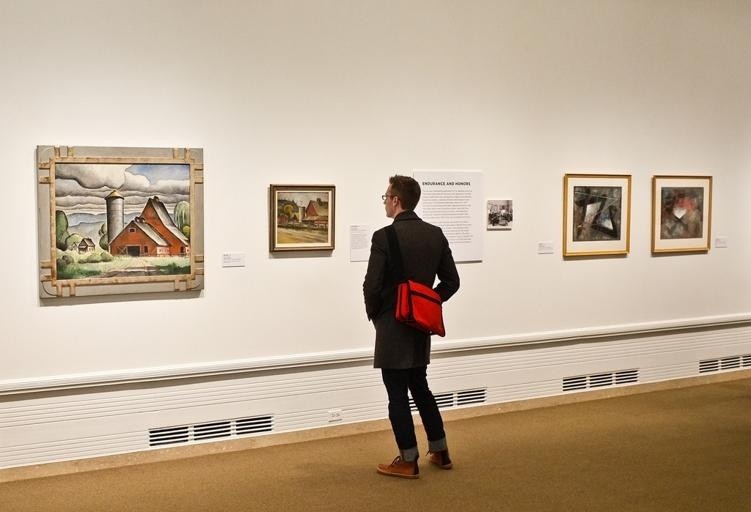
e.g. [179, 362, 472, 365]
[381, 194, 392, 201]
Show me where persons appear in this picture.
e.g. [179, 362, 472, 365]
[364, 174, 461, 478]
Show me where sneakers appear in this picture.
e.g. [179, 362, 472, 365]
[377, 456, 420, 479]
[428, 451, 453, 469]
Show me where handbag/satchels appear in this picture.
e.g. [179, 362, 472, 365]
[395, 280, 445, 337]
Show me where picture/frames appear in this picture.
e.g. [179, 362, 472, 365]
[35, 146, 204, 300]
[562, 174, 631, 258]
[269, 184, 336, 252]
[652, 175, 712, 253]
